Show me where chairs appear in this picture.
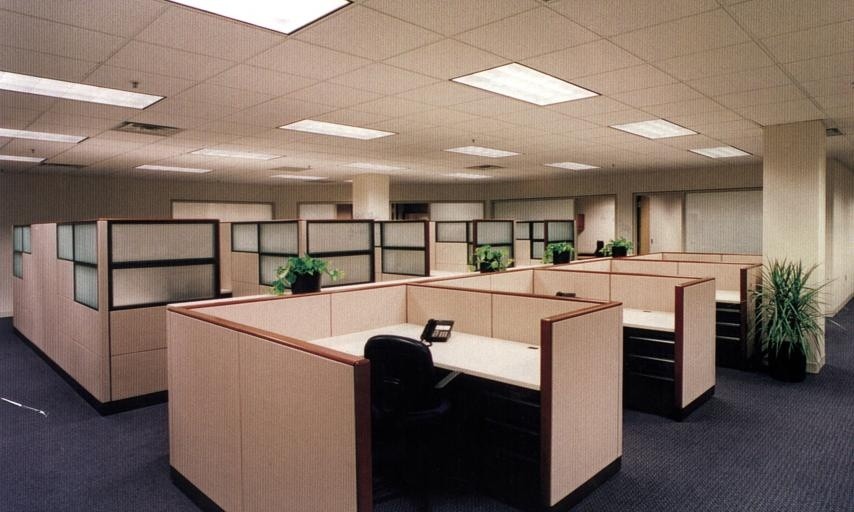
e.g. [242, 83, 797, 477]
[363, 339, 489, 512]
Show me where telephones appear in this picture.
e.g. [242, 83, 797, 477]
[420, 319, 454, 342]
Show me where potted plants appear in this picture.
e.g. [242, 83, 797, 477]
[268, 253, 346, 297]
[599, 235, 635, 256]
[539, 242, 576, 266]
[468, 245, 516, 274]
[743, 253, 848, 384]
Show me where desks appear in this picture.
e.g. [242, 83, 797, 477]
[715, 290, 741, 368]
[623, 305, 676, 415]
[303, 323, 541, 512]
